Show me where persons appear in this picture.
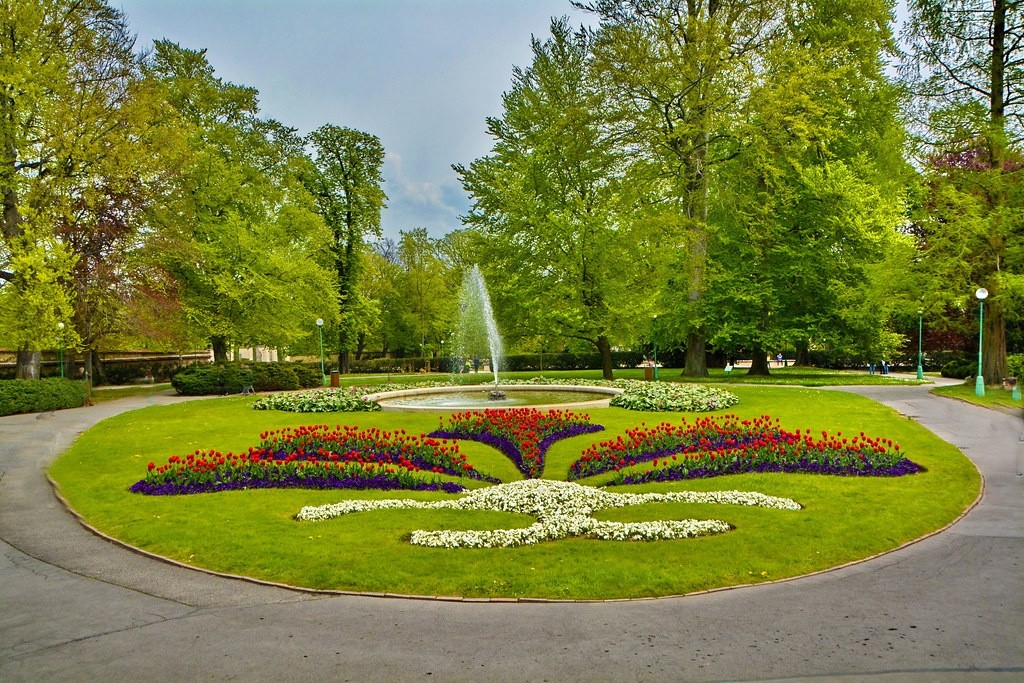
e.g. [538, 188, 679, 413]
[217, 365, 228, 397]
[459, 357, 465, 373]
[474, 355, 479, 373]
[238, 363, 256, 396]
[767, 354, 771, 368]
[869, 360, 890, 374]
[777, 353, 783, 366]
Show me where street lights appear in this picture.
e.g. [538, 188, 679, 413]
[315, 318, 328, 387]
[917, 306, 925, 380]
[653, 314, 659, 380]
[975, 288, 989, 396]
[56, 323, 65, 379]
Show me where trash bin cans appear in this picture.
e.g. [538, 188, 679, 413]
[644, 364, 654, 380]
[330, 371, 339, 386]
